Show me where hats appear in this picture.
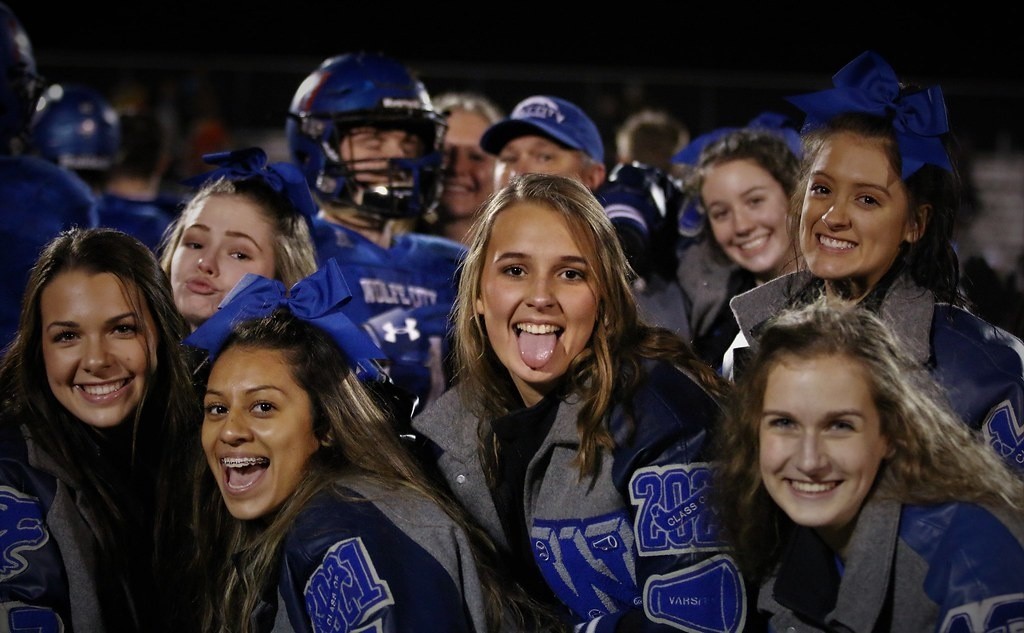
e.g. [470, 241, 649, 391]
[479, 95, 604, 164]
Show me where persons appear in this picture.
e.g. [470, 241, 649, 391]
[716, 292, 1024, 633]
[0, 227, 215, 633]
[411, 175, 753, 633]
[185, 257, 566, 633]
[0, 16, 1024, 455]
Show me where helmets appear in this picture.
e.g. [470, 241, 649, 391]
[285, 49, 449, 221]
[28, 83, 135, 169]
